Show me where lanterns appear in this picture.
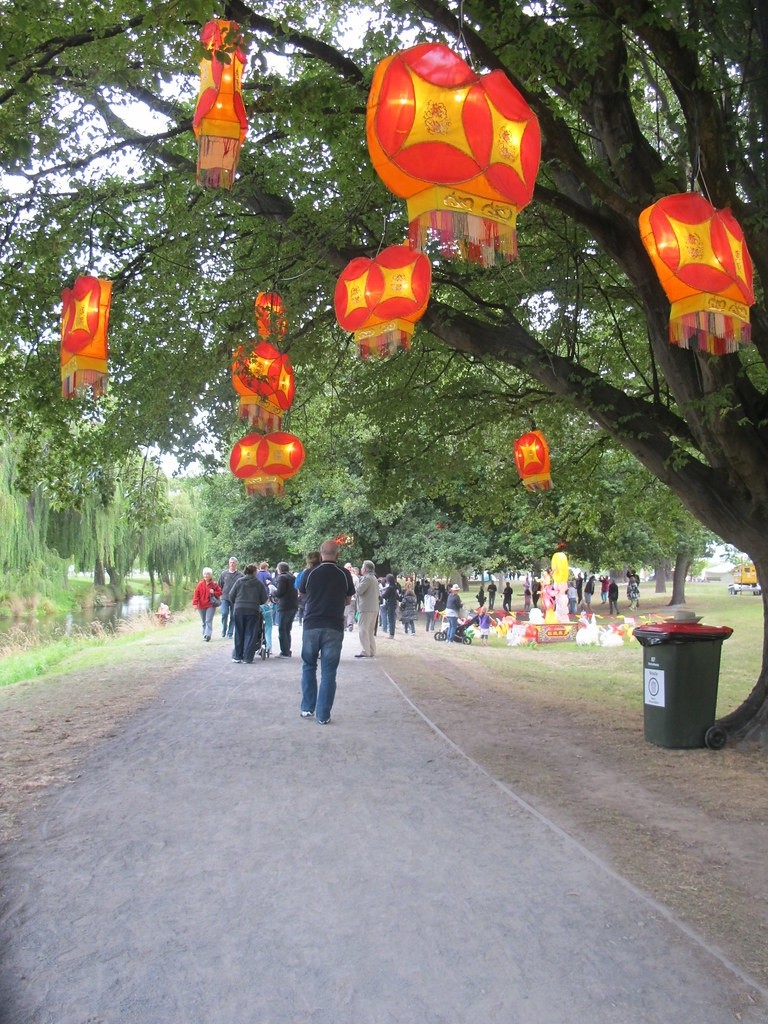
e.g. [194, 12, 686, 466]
[334, 246, 432, 359]
[513, 430, 555, 492]
[60, 277, 113, 401]
[636, 193, 755, 356]
[230, 432, 306, 497]
[333, 534, 353, 545]
[254, 291, 289, 344]
[365, 44, 542, 268]
[231, 340, 295, 432]
[192, 19, 248, 187]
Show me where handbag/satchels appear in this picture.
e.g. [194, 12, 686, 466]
[209, 583, 222, 607]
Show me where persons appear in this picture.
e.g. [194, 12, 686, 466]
[218, 558, 243, 638]
[476, 579, 541, 646]
[255, 552, 323, 667]
[229, 563, 269, 663]
[192, 567, 223, 642]
[297, 540, 356, 725]
[566, 569, 640, 615]
[342, 560, 463, 659]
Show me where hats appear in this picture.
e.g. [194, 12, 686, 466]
[428, 588, 434, 595]
[451, 584, 460, 591]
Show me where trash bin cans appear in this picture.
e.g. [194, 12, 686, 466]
[632, 620, 735, 752]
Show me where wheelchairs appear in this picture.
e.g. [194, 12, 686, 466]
[260, 618, 270, 660]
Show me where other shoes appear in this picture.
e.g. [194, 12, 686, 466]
[355, 654, 374, 657]
[349, 624, 353, 632]
[318, 717, 331, 725]
[232, 658, 247, 664]
[629, 607, 633, 612]
[222, 634, 225, 637]
[446, 639, 454, 644]
[301, 710, 314, 719]
[388, 634, 394, 639]
[275, 653, 291, 659]
[411, 633, 415, 636]
[204, 635, 210, 642]
[228, 634, 232, 639]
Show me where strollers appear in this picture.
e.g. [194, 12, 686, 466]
[433, 613, 479, 645]
